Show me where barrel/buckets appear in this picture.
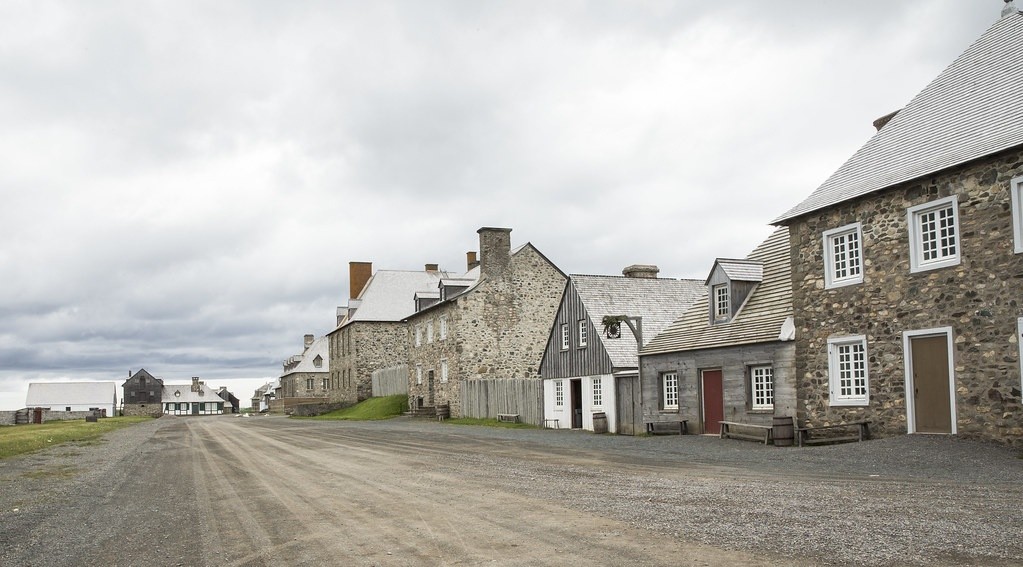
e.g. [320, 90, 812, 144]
[593, 412, 608, 434]
[772, 417, 794, 447]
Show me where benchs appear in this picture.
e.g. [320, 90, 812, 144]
[718, 421, 773, 445]
[543, 418, 559, 430]
[794, 420, 871, 448]
[643, 420, 689, 435]
[496, 413, 518, 423]
